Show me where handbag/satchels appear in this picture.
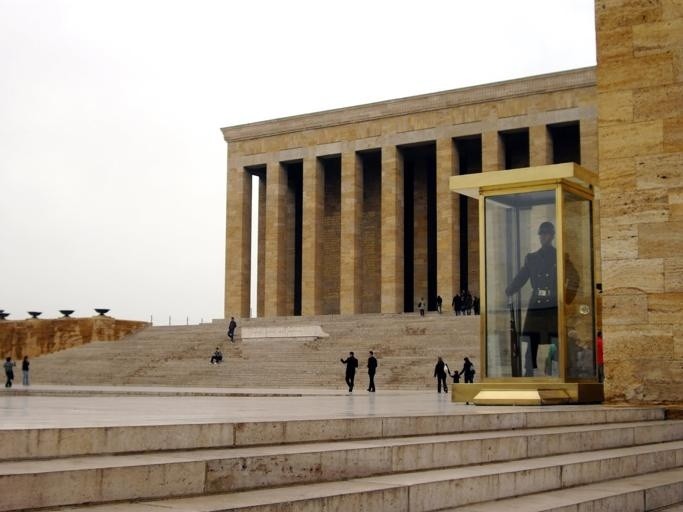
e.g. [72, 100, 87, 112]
[444, 364, 448, 374]
[469, 365, 475, 374]
[418, 302, 421, 307]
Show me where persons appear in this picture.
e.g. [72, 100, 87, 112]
[460, 358, 475, 383]
[22, 356, 29, 385]
[545, 332, 603, 383]
[228, 317, 236, 343]
[418, 298, 426, 317]
[450, 371, 461, 383]
[367, 351, 377, 392]
[434, 357, 450, 393]
[435, 290, 480, 316]
[4, 357, 16, 387]
[340, 352, 358, 392]
[209, 347, 222, 364]
[505, 222, 580, 377]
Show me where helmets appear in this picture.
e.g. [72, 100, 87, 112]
[538, 221, 554, 235]
